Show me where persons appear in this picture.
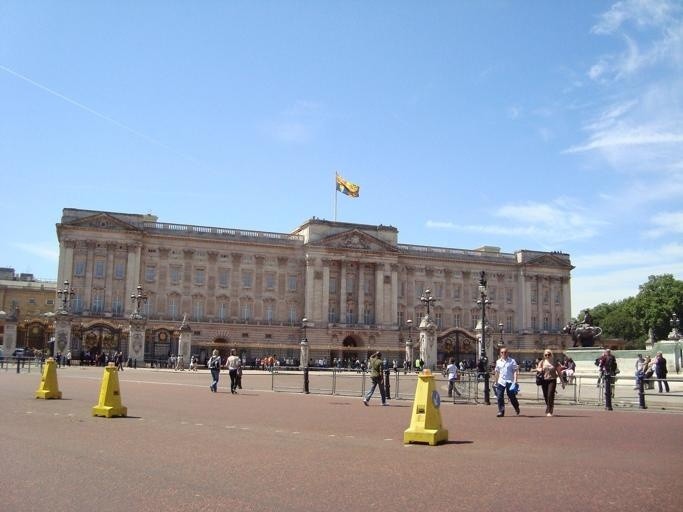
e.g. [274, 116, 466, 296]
[362, 351, 390, 406]
[634, 351, 669, 393]
[256, 354, 279, 370]
[175, 355, 184, 371]
[66, 350, 72, 367]
[334, 357, 368, 374]
[80, 352, 124, 371]
[443, 357, 461, 397]
[459, 360, 474, 374]
[207, 349, 221, 392]
[495, 348, 520, 416]
[536, 349, 565, 416]
[595, 349, 617, 396]
[190, 356, 197, 372]
[56, 352, 61, 368]
[393, 359, 425, 373]
[226, 349, 241, 393]
[556, 357, 576, 384]
[580, 310, 593, 326]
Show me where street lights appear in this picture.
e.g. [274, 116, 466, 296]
[406, 319, 414, 341]
[475, 270, 491, 379]
[301, 317, 309, 342]
[420, 289, 438, 315]
[57, 280, 76, 313]
[129, 284, 148, 320]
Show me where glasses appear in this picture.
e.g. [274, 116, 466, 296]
[545, 354, 551, 357]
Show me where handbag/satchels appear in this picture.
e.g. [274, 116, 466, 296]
[536, 373, 544, 386]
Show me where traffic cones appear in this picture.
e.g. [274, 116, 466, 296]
[401, 369, 446, 446]
[34, 358, 62, 401]
[93, 363, 127, 418]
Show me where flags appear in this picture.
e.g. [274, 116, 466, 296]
[336, 172, 360, 199]
